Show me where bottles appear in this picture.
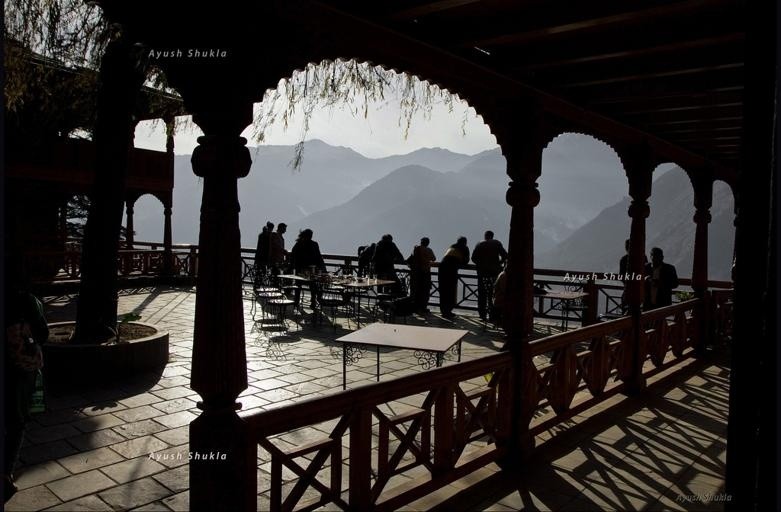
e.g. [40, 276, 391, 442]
[31, 369, 47, 413]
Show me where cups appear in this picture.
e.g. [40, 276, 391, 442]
[352, 272, 378, 284]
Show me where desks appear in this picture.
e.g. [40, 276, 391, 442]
[335, 322, 470, 390]
[533, 291, 590, 331]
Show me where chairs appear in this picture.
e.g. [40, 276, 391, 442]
[246, 263, 410, 341]
[482, 275, 512, 337]
[561, 279, 589, 332]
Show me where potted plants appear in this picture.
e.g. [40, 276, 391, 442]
[675, 291, 695, 316]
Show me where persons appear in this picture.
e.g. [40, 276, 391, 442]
[407, 238, 435, 315]
[471, 231, 507, 320]
[645, 247, 677, 330]
[357, 243, 375, 281]
[491, 262, 546, 315]
[436, 235, 470, 318]
[1, 290, 48, 512]
[253, 221, 276, 292]
[290, 227, 327, 309]
[373, 234, 405, 299]
[269, 223, 287, 287]
[618, 237, 649, 315]
